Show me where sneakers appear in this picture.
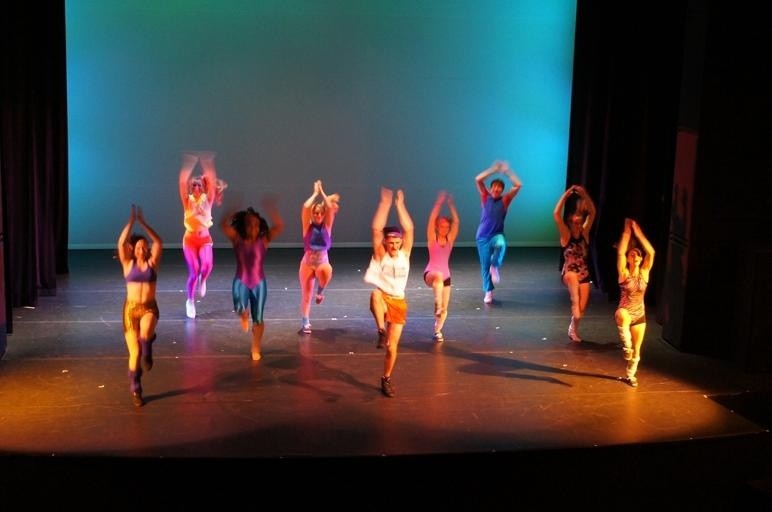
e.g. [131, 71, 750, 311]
[186, 271, 208, 319]
[251, 345, 262, 361]
[303, 291, 324, 335]
[623, 346, 639, 388]
[142, 352, 154, 372]
[240, 310, 249, 333]
[433, 302, 444, 342]
[381, 374, 397, 399]
[483, 264, 501, 305]
[376, 327, 388, 350]
[568, 303, 584, 342]
[132, 385, 143, 407]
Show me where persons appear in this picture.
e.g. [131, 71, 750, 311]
[299, 180, 340, 334]
[424, 192, 460, 343]
[615, 218, 656, 387]
[118, 204, 161, 406]
[475, 162, 521, 303]
[554, 185, 596, 342]
[223, 201, 283, 361]
[363, 187, 414, 398]
[179, 150, 219, 318]
